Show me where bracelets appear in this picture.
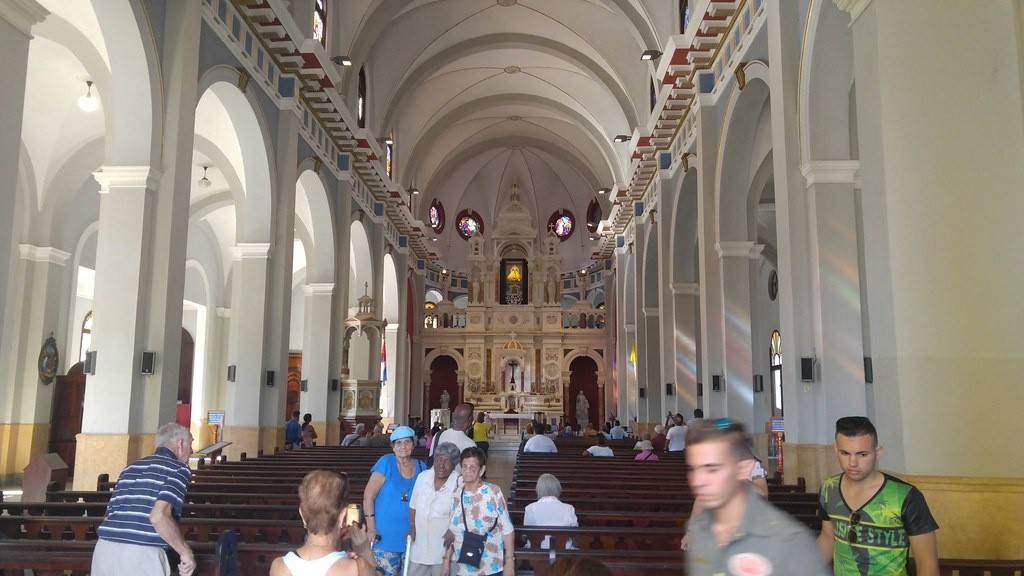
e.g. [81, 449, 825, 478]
[364, 514, 375, 517]
[505, 555, 515, 561]
[410, 522, 415, 527]
[663, 448, 668, 451]
[443, 555, 451, 560]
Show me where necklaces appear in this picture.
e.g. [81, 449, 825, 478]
[399, 460, 412, 501]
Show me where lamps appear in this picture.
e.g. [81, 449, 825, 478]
[581, 269, 585, 273]
[587, 222, 598, 227]
[640, 49, 663, 61]
[427, 237, 438, 242]
[598, 188, 612, 194]
[78, 81, 98, 112]
[614, 135, 632, 143]
[198, 166, 212, 188]
[589, 237, 600, 241]
[442, 268, 446, 273]
[329, 56, 352, 66]
[405, 188, 420, 195]
[375, 137, 394, 145]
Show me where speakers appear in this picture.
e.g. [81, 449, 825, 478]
[696, 383, 702, 395]
[300, 380, 306, 391]
[800, 356, 814, 382]
[265, 370, 275, 387]
[227, 365, 236, 381]
[711, 375, 720, 392]
[331, 378, 339, 391]
[140, 350, 155, 375]
[755, 375, 763, 392]
[666, 383, 671, 396]
[639, 387, 644, 398]
[83, 350, 97, 375]
[864, 357, 873, 383]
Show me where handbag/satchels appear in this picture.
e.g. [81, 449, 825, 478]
[426, 455, 434, 469]
[467, 427, 473, 439]
[456, 531, 488, 568]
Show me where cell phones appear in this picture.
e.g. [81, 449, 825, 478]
[346, 503, 362, 530]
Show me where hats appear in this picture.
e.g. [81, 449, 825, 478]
[389, 426, 415, 442]
[640, 440, 654, 451]
[565, 426, 572, 431]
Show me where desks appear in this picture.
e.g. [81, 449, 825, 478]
[488, 412, 534, 435]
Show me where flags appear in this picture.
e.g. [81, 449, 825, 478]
[380, 325, 387, 385]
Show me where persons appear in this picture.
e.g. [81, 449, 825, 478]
[440, 390, 450, 407]
[524, 425, 557, 452]
[270, 470, 382, 576]
[634, 408, 705, 454]
[91, 421, 197, 576]
[522, 419, 629, 440]
[815, 416, 939, 576]
[523, 474, 578, 569]
[364, 402, 515, 576]
[284, 411, 317, 450]
[635, 440, 659, 461]
[341, 423, 371, 446]
[576, 391, 590, 428]
[582, 436, 614, 456]
[680, 417, 831, 576]
[432, 411, 438, 427]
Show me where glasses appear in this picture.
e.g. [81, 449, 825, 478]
[685, 417, 763, 463]
[847, 511, 862, 544]
[461, 401, 474, 419]
[322, 468, 348, 508]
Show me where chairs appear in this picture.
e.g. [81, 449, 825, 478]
[503, 409, 520, 434]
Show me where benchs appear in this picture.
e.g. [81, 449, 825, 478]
[503, 437, 822, 576]
[0, 442, 428, 576]
